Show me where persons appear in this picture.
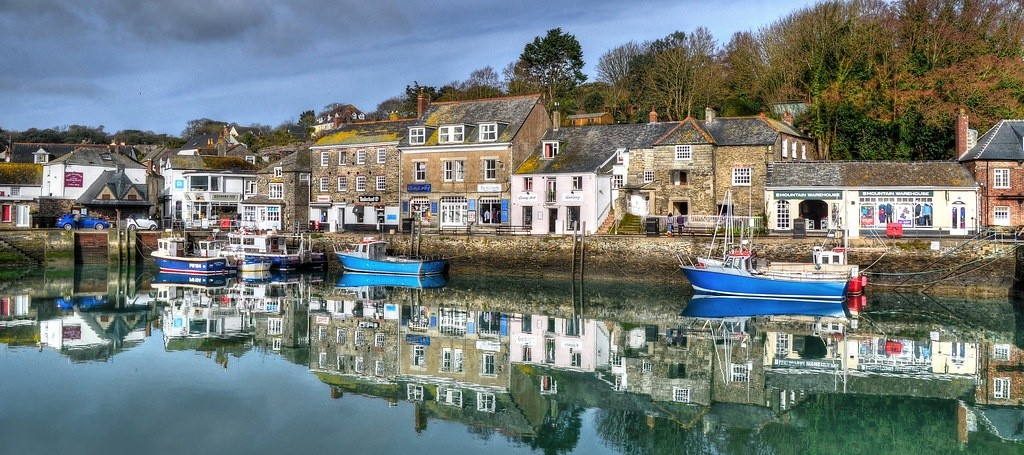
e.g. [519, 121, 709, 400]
[666, 213, 674, 237]
[677, 214, 684, 237]
[484, 209, 489, 220]
[493, 208, 501, 223]
[74, 211, 80, 230]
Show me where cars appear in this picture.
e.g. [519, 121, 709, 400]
[54, 213, 110, 231]
[126, 213, 159, 232]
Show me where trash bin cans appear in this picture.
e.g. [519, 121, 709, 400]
[402, 218, 415, 234]
[835, 230, 842, 238]
[792, 334, 805, 351]
[646, 217, 659, 236]
[645, 325, 658, 342]
[402, 305, 411, 319]
[32, 217, 54, 228]
[793, 218, 805, 238]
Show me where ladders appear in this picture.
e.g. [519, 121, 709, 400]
[572, 221, 585, 278]
[409, 288, 420, 323]
[292, 220, 300, 246]
[409, 221, 422, 260]
[571, 278, 585, 336]
[291, 283, 299, 310]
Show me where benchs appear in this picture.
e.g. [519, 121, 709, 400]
[671, 227, 715, 238]
[495, 225, 532, 236]
[439, 226, 471, 236]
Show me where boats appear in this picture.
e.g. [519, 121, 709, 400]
[332, 236, 449, 274]
[148, 199, 329, 276]
[676, 292, 867, 399]
[675, 187, 868, 300]
[333, 269, 450, 303]
